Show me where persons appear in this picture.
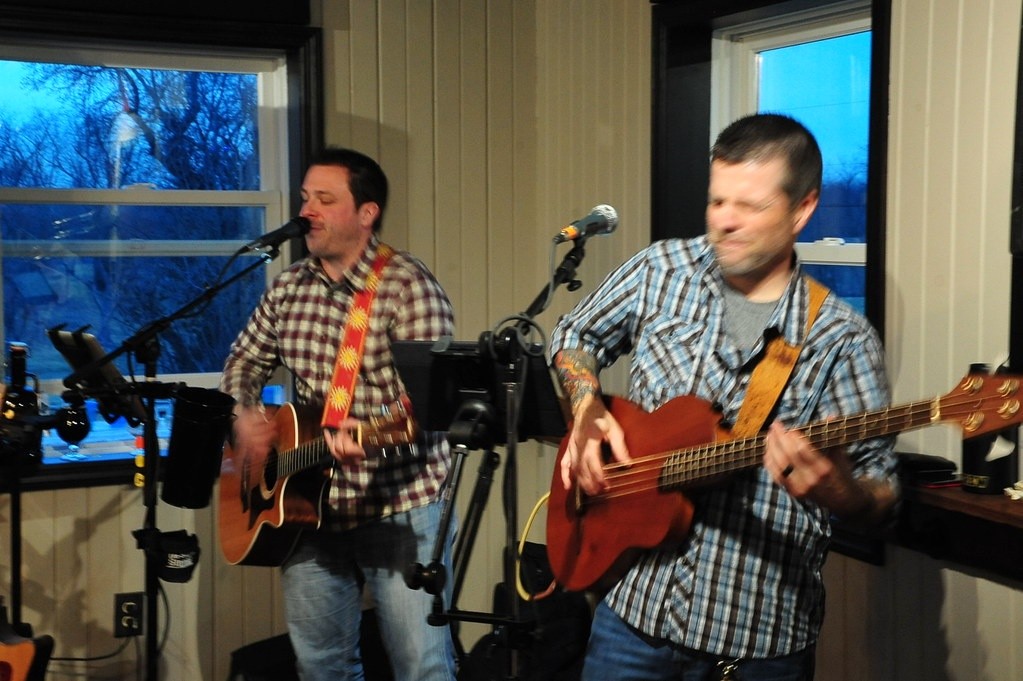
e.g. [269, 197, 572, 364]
[219, 147, 458, 681]
[546, 115, 900, 681]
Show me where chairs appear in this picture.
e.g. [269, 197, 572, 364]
[455, 541, 593, 681]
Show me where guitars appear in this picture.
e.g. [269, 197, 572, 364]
[216, 393, 418, 568]
[1, 382, 56, 681]
[544, 373, 1023, 598]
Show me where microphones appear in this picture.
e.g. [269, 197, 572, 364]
[553, 204, 619, 244]
[241, 216, 311, 253]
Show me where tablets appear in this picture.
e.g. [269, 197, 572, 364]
[392, 339, 568, 443]
[50, 329, 151, 425]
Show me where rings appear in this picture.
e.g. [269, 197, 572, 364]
[781, 465, 793, 478]
